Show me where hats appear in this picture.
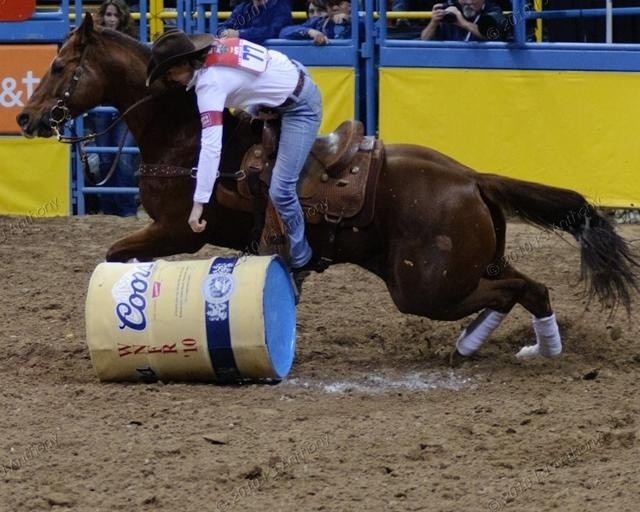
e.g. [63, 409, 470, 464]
[145, 29, 213, 87]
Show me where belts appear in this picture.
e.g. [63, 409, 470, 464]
[279, 69, 304, 107]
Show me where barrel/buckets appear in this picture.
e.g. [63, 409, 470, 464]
[85, 255, 298, 381]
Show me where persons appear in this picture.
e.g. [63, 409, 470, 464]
[391, 1, 412, 28]
[214, 1, 296, 43]
[305, 1, 328, 20]
[278, 1, 350, 46]
[90, 1, 139, 218]
[166, 38, 322, 275]
[420, 0, 510, 41]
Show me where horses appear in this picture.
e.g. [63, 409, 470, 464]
[13, 8, 640, 369]
